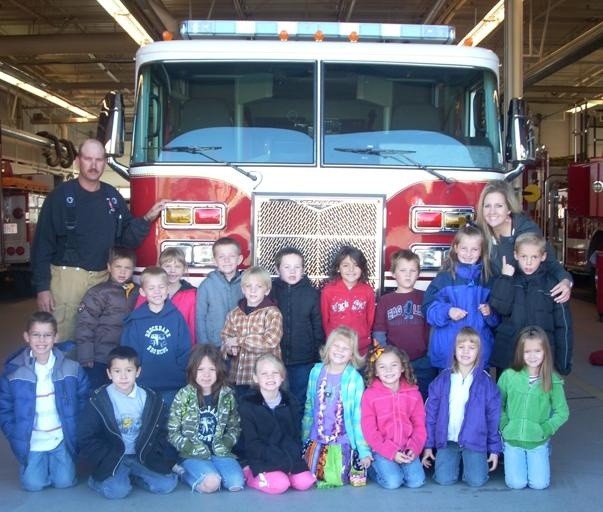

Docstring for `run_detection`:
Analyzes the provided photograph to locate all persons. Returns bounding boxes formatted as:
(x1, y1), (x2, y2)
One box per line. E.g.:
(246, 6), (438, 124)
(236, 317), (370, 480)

(30, 139), (172, 346)
(0, 312), (93, 492)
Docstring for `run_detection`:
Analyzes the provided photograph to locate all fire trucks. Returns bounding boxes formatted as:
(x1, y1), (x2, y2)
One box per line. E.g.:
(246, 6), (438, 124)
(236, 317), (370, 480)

(535, 158), (603, 304)
(0, 160), (65, 283)
(93, 18), (532, 296)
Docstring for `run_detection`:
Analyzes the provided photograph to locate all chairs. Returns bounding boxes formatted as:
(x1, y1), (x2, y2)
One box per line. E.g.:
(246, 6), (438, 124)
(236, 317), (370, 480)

(390, 103), (443, 135)
(169, 99), (228, 143)
